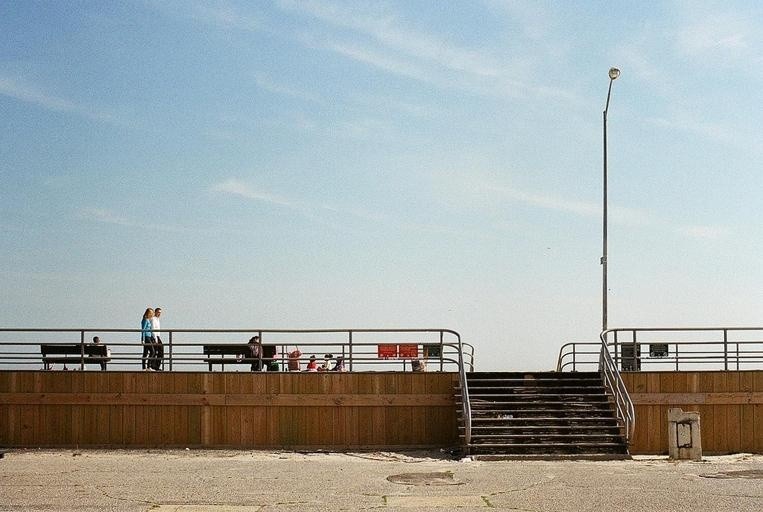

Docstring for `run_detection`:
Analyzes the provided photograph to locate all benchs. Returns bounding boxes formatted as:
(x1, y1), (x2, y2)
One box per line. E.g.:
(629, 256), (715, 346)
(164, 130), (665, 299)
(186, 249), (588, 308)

(202, 343), (276, 372)
(39, 342), (111, 371)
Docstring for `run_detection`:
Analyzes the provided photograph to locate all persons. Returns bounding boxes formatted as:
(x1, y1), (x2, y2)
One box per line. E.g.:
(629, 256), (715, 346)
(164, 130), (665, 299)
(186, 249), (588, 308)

(140, 308), (156, 371)
(317, 354), (331, 372)
(149, 307), (164, 371)
(330, 356), (343, 370)
(87, 336), (111, 371)
(244, 337), (255, 358)
(305, 354), (316, 372)
(247, 336), (264, 372)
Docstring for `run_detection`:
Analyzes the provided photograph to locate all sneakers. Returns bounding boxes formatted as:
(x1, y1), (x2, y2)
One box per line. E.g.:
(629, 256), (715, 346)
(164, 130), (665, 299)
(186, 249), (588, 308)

(142, 367), (161, 372)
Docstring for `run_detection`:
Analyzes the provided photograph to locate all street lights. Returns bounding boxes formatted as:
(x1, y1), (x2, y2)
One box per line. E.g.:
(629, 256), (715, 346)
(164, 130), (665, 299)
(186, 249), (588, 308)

(593, 62), (624, 374)
